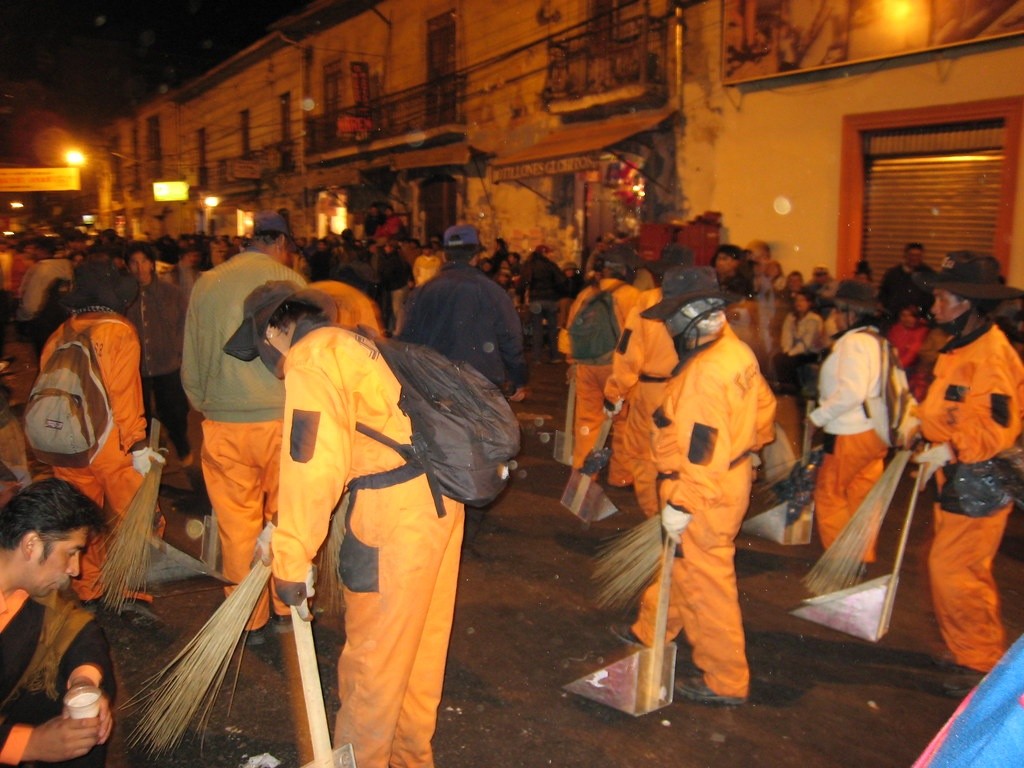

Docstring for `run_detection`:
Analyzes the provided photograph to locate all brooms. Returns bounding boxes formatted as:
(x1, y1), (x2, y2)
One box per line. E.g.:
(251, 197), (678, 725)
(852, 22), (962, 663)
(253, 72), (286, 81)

(319, 492), (351, 599)
(761, 421), (796, 484)
(799, 450), (911, 596)
(91, 417), (165, 615)
(590, 513), (663, 609)
(116, 556), (272, 762)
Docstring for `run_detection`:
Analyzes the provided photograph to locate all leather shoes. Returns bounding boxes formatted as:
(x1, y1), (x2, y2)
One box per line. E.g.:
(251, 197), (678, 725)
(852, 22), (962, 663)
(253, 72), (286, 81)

(676, 675), (747, 706)
(610, 624), (644, 647)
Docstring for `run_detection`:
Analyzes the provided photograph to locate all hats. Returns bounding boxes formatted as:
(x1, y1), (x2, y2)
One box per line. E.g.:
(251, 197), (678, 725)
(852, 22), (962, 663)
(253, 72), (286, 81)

(641, 264), (736, 319)
(245, 211), (295, 244)
(644, 243), (695, 278)
(443, 224), (488, 253)
(818, 280), (882, 317)
(226, 279), (326, 360)
(921, 250), (1024, 302)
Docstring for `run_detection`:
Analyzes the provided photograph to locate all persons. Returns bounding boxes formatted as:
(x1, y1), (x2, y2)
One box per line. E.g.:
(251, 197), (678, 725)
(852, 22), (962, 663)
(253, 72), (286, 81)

(800, 281), (909, 576)
(0, 478), (117, 768)
(0, 223), (309, 346)
(122, 243), (191, 470)
(888, 304), (927, 375)
(706, 240), (882, 406)
(476, 230), (694, 518)
(877, 242), (938, 320)
(614, 264), (777, 704)
(42, 253), (164, 608)
(911, 252), (1024, 695)
(0, 395), (31, 492)
(391, 225), (527, 403)
(220, 282), (465, 767)
(179, 216), (309, 645)
(297, 201), (447, 334)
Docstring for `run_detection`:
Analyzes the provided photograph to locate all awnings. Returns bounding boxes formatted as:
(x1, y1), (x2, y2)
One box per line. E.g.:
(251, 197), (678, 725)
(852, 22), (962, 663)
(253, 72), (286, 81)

(306, 144), (494, 218)
(493, 105), (675, 207)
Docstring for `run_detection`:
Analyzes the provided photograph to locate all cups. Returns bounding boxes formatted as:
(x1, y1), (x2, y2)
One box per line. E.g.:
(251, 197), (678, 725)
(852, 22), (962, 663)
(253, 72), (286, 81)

(62, 684), (103, 719)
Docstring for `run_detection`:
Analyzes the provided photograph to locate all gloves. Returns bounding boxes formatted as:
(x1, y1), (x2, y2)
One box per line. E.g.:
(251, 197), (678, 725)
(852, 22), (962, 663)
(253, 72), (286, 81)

(602, 398), (624, 420)
(910, 445), (953, 492)
(660, 506), (692, 542)
(293, 569), (315, 621)
(251, 521), (277, 569)
(130, 446), (167, 477)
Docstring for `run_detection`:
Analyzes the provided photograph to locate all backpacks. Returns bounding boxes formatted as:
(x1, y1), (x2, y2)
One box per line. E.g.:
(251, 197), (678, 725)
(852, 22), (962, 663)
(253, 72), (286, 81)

(570, 280), (629, 359)
(24, 315), (113, 466)
(297, 324), (522, 508)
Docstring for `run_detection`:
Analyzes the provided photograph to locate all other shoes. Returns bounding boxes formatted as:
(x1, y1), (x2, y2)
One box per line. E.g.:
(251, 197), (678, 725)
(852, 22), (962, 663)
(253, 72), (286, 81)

(943, 666), (986, 689)
(99, 595), (165, 633)
(605, 482), (635, 496)
(179, 451), (194, 468)
